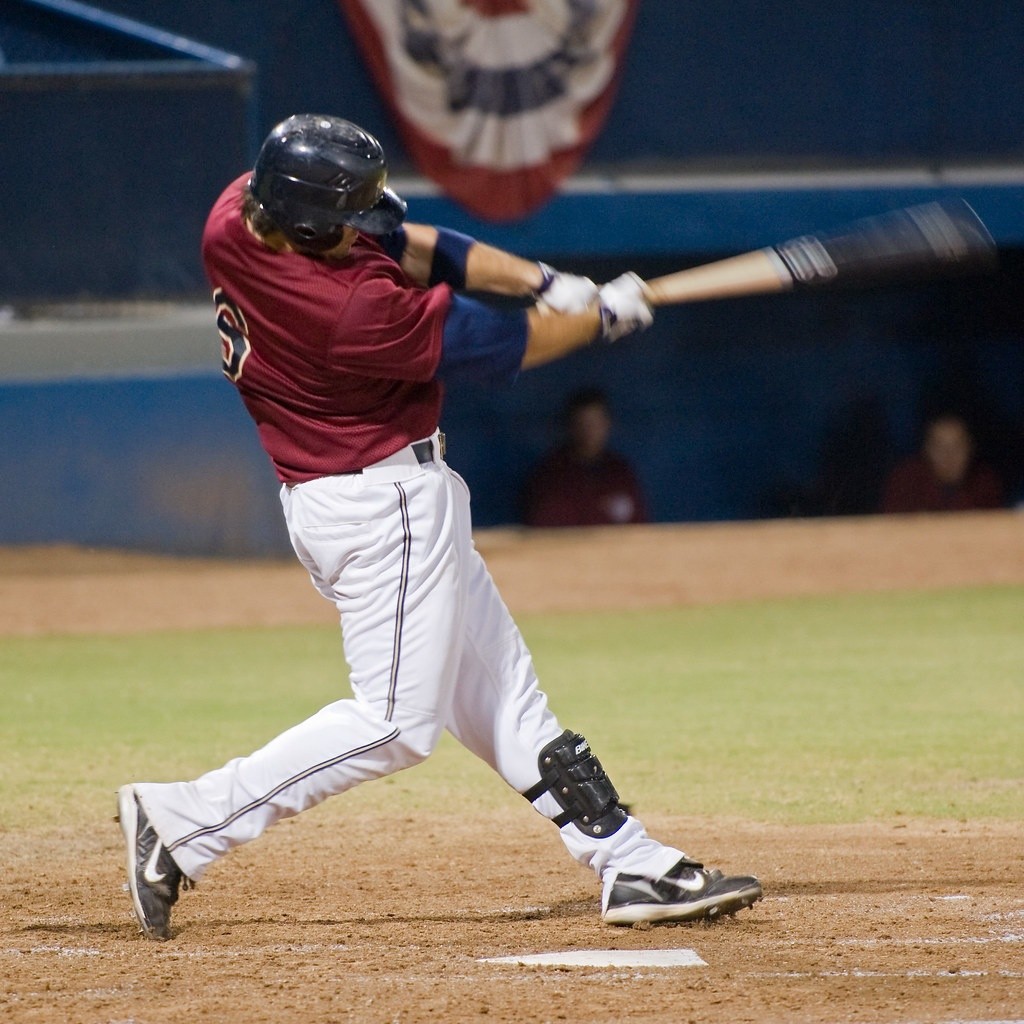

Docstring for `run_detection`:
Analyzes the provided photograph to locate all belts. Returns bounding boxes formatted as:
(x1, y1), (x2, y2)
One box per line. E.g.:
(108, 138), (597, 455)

(341, 432), (449, 475)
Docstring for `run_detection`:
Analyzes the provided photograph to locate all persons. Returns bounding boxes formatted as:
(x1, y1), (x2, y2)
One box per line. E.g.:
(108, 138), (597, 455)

(522, 387), (649, 526)
(877, 403), (1003, 513)
(117, 114), (763, 941)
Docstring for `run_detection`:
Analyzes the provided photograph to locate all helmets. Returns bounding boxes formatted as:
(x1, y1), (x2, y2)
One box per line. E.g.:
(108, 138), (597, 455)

(248, 112), (409, 251)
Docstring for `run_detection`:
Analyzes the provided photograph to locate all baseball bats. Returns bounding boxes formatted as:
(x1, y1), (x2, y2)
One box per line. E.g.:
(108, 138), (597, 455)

(646, 183), (1024, 305)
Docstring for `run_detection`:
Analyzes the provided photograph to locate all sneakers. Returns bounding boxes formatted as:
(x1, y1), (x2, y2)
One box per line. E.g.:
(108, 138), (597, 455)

(117, 784), (188, 941)
(598, 855), (762, 924)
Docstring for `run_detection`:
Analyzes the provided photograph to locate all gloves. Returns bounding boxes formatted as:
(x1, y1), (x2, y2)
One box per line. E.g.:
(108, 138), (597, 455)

(597, 269), (656, 343)
(533, 258), (598, 317)
